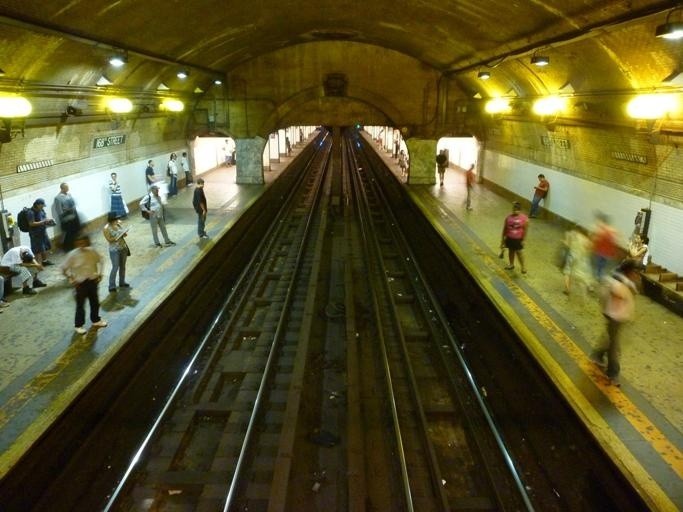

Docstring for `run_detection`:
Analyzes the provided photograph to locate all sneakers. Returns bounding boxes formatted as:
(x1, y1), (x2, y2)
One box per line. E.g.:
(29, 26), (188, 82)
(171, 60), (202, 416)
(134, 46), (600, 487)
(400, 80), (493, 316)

(34, 280), (46, 287)
(23, 286), (36, 294)
(42, 259), (54, 265)
(75, 327), (86, 334)
(92, 321), (107, 327)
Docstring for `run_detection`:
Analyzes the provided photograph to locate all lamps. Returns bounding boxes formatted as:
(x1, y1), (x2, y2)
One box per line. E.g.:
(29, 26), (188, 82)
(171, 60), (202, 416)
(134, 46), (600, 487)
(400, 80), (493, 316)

(529, 44), (549, 67)
(654, 7), (682, 40)
(109, 42), (128, 65)
(477, 61), (490, 79)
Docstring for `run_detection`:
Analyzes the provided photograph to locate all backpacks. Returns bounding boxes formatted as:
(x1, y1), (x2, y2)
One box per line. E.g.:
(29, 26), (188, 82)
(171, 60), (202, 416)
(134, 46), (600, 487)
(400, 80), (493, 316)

(18, 207), (29, 232)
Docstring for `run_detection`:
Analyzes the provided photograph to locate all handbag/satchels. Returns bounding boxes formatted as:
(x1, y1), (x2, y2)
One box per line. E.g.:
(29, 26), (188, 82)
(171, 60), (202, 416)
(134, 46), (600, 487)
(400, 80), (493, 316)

(142, 205), (149, 219)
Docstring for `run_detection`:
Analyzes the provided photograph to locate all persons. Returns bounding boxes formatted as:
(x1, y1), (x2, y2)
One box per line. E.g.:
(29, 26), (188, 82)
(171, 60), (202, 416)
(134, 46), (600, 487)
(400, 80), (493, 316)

(320, 125), (324, 141)
(395, 141), (400, 158)
(222, 139), (232, 167)
(501, 202), (528, 274)
(464, 164), (476, 210)
(559, 216), (649, 387)
(286, 137), (290, 154)
(398, 150), (407, 175)
(436, 149), (447, 185)
(0, 153), (208, 335)
(529, 174), (550, 218)
(378, 130), (384, 148)
(300, 129), (303, 142)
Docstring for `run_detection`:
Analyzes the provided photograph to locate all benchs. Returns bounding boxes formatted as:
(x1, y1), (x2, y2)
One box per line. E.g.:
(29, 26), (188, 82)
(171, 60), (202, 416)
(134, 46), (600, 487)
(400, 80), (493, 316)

(0, 265), (19, 297)
(638, 263), (682, 317)
